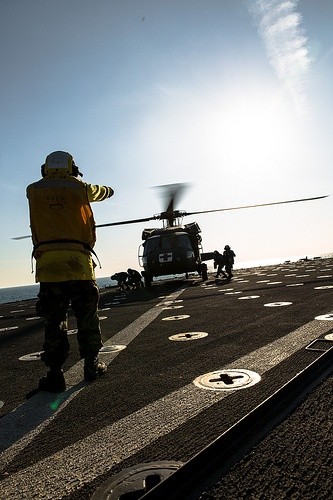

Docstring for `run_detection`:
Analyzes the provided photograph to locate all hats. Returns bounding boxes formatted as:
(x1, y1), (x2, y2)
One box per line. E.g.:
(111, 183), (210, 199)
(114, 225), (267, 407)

(43, 151), (75, 176)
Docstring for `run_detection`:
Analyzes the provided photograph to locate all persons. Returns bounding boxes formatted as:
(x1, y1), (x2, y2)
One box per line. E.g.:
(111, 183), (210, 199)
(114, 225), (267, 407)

(213, 250), (228, 278)
(25, 151), (114, 393)
(110, 272), (130, 291)
(127, 268), (143, 290)
(222, 245), (236, 278)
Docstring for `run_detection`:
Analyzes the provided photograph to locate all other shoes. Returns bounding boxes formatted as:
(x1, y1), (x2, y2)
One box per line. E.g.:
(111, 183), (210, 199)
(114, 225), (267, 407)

(37, 367), (66, 394)
(82, 358), (108, 383)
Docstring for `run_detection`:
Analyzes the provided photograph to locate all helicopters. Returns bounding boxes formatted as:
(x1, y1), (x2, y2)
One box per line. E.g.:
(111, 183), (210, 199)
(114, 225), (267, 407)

(12, 182), (329, 289)
